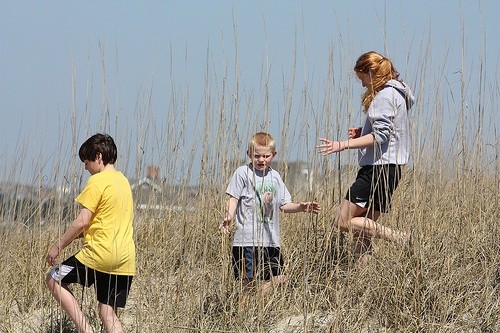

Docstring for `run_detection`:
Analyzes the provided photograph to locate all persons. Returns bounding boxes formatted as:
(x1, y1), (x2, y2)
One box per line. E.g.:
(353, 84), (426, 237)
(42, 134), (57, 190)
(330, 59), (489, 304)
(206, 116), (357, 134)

(46, 134), (136, 333)
(315, 52), (414, 268)
(219, 133), (320, 294)
